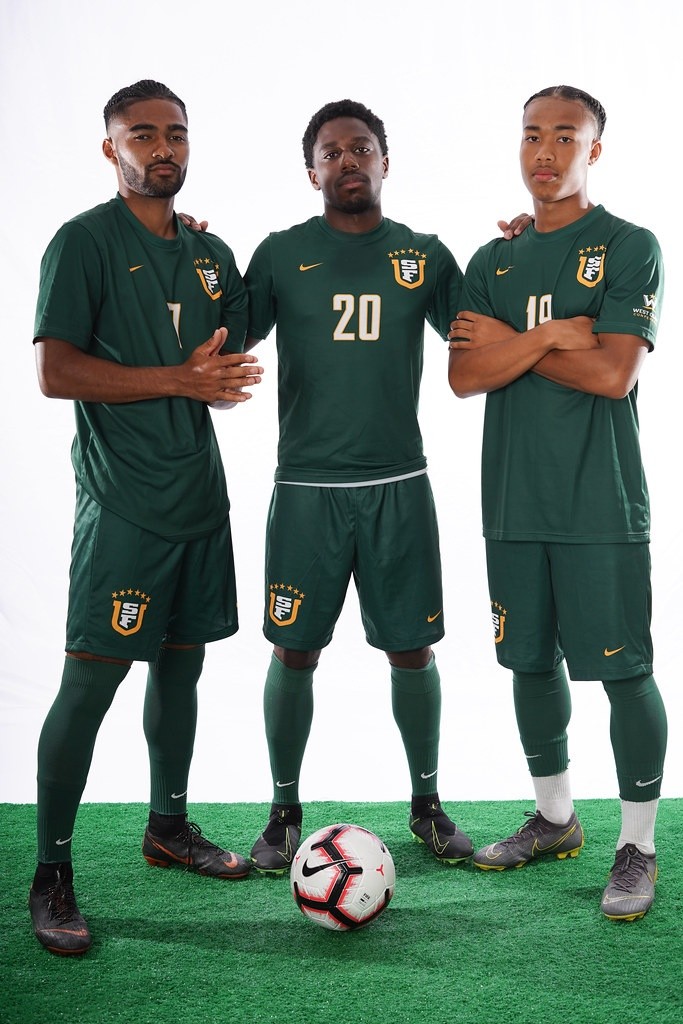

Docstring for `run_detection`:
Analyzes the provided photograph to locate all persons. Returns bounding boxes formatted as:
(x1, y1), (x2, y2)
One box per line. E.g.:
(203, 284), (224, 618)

(173, 97), (532, 876)
(27, 79), (254, 952)
(445, 83), (666, 923)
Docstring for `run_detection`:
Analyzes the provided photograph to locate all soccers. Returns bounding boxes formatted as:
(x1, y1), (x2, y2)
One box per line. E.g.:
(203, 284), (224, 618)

(291, 823), (399, 931)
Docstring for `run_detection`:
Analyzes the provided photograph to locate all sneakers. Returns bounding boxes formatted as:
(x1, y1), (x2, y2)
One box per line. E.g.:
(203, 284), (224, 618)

(599, 843), (657, 921)
(141, 821), (250, 878)
(472, 809), (583, 871)
(408, 793), (474, 865)
(250, 803), (302, 875)
(28, 864), (91, 956)
